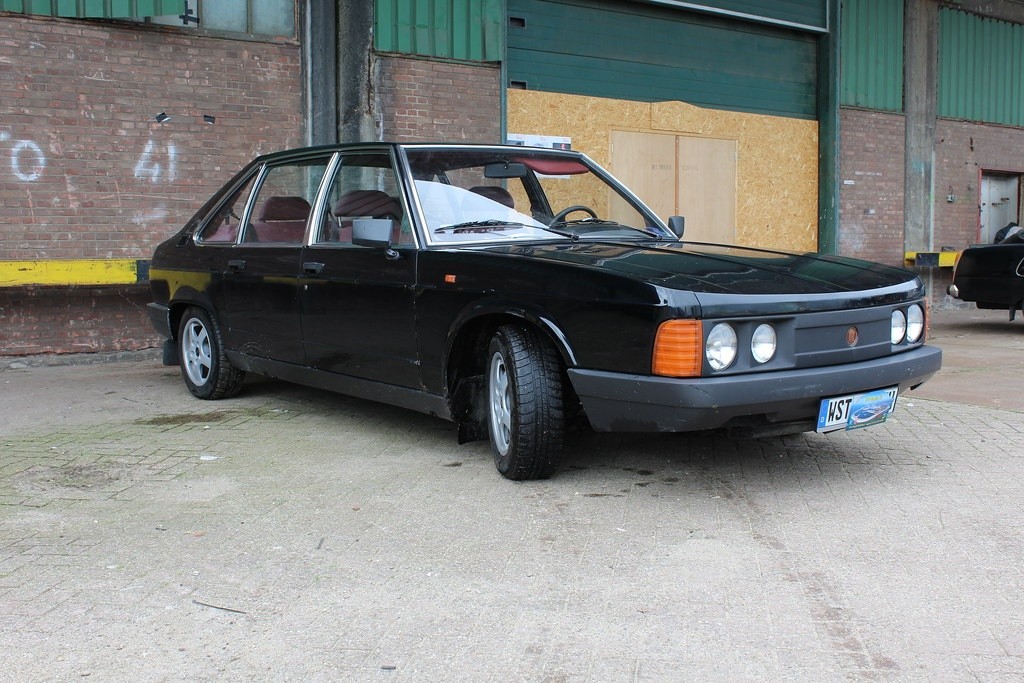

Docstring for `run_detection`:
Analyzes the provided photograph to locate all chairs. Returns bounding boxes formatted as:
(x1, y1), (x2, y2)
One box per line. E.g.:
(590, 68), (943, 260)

(452, 186), (524, 243)
(205, 191), (405, 246)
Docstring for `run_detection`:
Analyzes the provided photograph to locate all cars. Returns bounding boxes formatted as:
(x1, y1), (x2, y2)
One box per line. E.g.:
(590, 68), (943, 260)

(946, 243), (1024, 323)
(147, 140), (944, 482)
